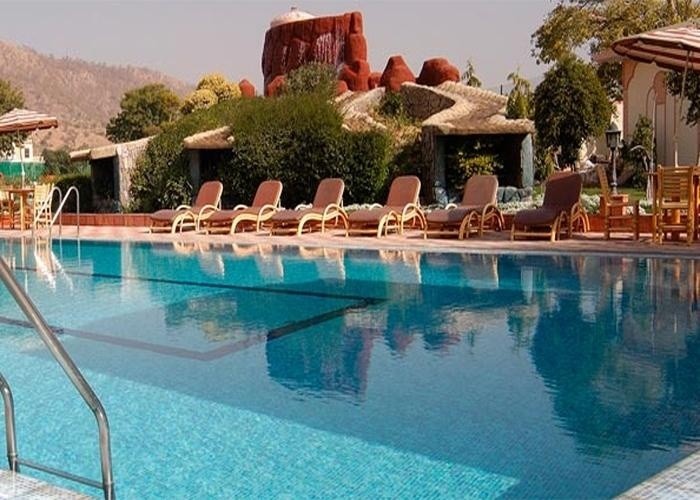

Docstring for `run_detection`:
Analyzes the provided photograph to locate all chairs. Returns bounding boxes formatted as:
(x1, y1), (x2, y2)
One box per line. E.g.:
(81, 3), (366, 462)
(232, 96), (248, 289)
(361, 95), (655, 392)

(462, 251), (499, 291)
(298, 245), (347, 289)
(150, 180), (224, 234)
(379, 249), (421, 300)
(174, 241), (225, 280)
(346, 175), (428, 240)
(596, 164), (700, 247)
(515, 254), (699, 325)
(232, 242), (285, 284)
(509, 172), (590, 242)
(269, 178), (350, 237)
(0, 237), (74, 290)
(206, 180), (284, 234)
(0, 182), (55, 230)
(424, 175), (506, 240)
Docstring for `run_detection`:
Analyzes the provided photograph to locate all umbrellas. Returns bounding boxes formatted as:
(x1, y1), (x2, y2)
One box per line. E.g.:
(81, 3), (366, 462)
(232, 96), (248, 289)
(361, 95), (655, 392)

(0, 107), (54, 171)
(610, 22), (699, 168)
